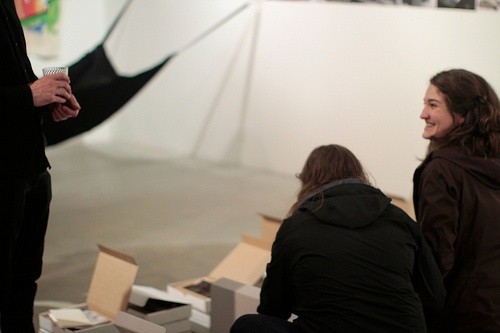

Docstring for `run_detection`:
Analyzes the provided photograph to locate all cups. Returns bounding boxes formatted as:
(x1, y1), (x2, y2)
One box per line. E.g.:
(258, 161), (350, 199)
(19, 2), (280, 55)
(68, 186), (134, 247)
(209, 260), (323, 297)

(42, 65), (72, 112)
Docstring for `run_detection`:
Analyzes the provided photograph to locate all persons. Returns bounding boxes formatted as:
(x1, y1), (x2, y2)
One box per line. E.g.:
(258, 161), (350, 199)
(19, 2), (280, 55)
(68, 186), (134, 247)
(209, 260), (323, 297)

(0, 0), (81, 333)
(413, 69), (500, 333)
(228, 145), (427, 333)
